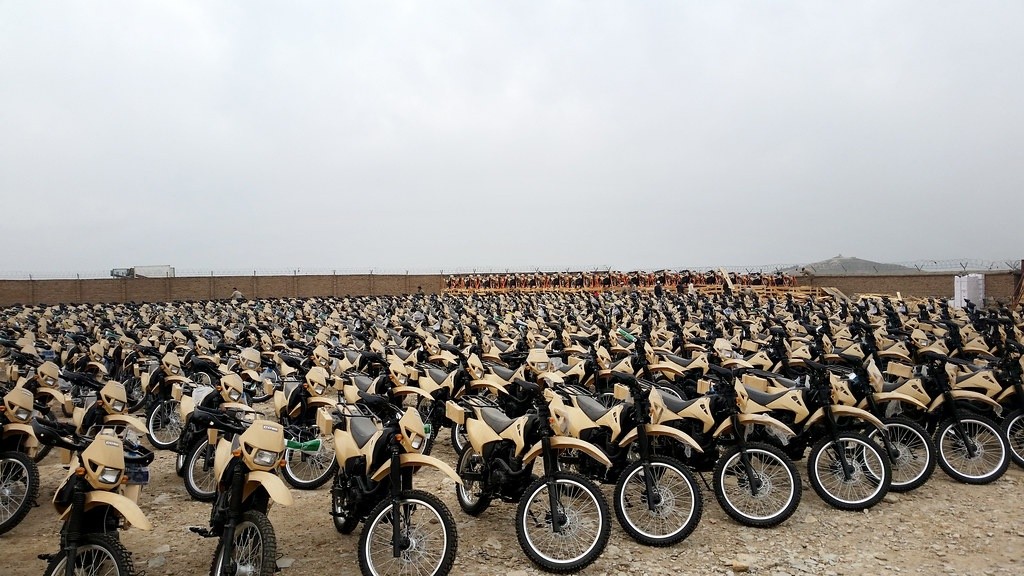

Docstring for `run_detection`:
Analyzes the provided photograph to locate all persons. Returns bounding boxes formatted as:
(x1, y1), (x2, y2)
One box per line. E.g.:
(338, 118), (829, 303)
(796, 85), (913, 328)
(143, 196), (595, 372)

(800, 268), (814, 286)
(745, 286), (754, 295)
(415, 286), (425, 297)
(723, 278), (728, 292)
(676, 282), (684, 293)
(228, 287), (244, 298)
(628, 282), (636, 293)
(620, 282), (627, 295)
(689, 280), (693, 292)
(654, 281), (663, 296)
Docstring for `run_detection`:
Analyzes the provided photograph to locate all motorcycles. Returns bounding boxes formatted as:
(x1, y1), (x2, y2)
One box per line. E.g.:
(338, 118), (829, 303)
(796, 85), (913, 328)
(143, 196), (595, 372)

(1, 264), (1024, 576)
